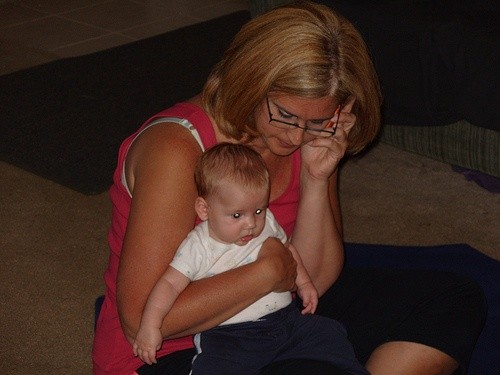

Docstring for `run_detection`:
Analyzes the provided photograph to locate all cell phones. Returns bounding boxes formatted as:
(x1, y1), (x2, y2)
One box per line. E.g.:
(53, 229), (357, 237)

(325, 105), (341, 129)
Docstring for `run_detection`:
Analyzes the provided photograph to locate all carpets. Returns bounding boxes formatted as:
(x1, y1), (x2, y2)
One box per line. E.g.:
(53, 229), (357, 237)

(0, 11), (252, 197)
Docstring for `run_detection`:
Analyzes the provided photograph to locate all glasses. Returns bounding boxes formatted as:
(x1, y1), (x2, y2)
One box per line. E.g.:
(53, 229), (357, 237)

(266, 94), (341, 138)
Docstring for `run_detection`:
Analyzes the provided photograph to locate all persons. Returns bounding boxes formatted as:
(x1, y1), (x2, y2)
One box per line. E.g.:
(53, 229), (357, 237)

(91, 5), (487, 375)
(132, 142), (370, 375)
